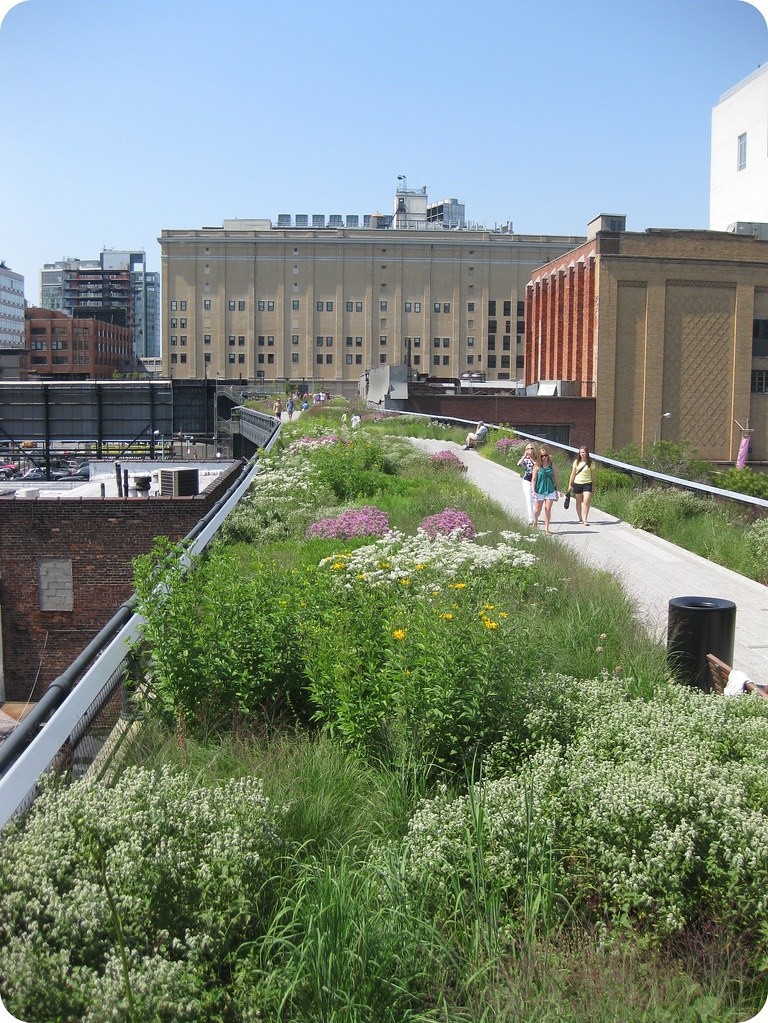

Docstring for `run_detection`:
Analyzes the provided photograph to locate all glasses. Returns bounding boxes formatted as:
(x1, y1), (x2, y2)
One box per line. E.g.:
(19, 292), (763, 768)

(541, 454), (549, 457)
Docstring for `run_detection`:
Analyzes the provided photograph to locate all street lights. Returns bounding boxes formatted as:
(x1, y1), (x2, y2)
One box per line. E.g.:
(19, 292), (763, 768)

(515, 381), (522, 395)
(153, 429), (164, 460)
(652, 412), (671, 470)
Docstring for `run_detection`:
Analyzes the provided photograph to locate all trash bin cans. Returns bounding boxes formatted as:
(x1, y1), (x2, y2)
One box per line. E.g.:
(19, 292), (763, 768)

(257, 370), (264, 377)
(666, 596), (737, 693)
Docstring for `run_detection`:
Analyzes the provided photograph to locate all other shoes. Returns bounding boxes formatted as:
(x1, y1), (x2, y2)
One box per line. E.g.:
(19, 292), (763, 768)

(463, 447), (469, 450)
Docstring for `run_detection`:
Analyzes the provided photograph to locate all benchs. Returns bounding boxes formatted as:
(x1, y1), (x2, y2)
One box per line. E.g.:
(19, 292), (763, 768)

(470, 432), (487, 449)
(705, 653), (768, 699)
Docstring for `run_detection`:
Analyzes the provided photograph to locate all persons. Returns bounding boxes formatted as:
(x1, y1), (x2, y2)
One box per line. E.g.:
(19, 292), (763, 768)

(293, 390), (330, 405)
(286, 398), (293, 421)
(516, 445), (538, 527)
(303, 401), (308, 410)
(273, 399), (283, 420)
(341, 412), (361, 428)
(216, 452), (221, 459)
(569, 446), (595, 526)
(532, 449), (563, 533)
(462, 421), (488, 450)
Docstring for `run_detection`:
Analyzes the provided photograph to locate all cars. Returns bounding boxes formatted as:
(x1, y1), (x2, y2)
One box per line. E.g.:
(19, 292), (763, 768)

(0, 451), (89, 482)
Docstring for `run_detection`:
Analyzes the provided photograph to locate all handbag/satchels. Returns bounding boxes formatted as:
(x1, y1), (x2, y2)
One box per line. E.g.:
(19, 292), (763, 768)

(564, 492), (570, 509)
